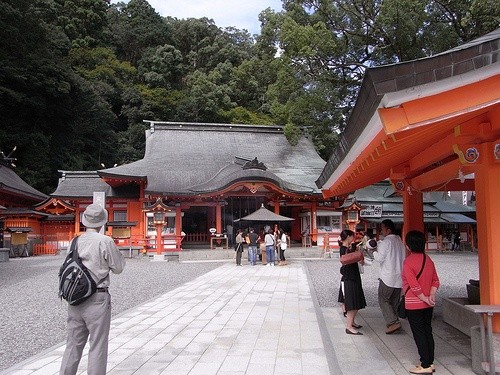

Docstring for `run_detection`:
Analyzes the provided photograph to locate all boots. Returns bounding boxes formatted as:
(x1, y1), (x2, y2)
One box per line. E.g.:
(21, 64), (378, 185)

(278, 261), (283, 265)
(283, 260), (287, 265)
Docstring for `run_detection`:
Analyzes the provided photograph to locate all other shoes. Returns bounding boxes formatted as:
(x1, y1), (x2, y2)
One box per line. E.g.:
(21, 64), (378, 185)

(352, 324), (363, 329)
(346, 328), (363, 335)
(266, 263), (270, 266)
(271, 263), (274, 266)
(344, 312), (347, 317)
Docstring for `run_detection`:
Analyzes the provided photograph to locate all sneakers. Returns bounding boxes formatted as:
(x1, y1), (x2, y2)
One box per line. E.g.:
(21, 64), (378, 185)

(409, 365), (432, 375)
(416, 363), (435, 372)
(385, 322), (400, 333)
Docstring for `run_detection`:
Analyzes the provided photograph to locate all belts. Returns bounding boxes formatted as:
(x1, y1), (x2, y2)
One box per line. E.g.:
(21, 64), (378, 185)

(97, 288), (105, 292)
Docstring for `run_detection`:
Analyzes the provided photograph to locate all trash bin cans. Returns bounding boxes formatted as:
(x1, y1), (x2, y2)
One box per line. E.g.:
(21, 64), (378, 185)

(465, 304), (500, 375)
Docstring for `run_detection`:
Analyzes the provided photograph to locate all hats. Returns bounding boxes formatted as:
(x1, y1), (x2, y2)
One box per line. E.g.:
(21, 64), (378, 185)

(82, 204), (108, 227)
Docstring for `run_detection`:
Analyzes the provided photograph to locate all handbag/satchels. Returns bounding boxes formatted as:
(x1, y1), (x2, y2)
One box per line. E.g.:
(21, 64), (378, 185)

(340, 246), (363, 265)
(397, 295), (407, 319)
(246, 233), (250, 243)
(58, 234), (97, 306)
(274, 242), (276, 250)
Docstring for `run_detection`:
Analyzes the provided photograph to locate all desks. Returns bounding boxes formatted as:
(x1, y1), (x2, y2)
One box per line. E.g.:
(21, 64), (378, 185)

(210, 236), (229, 252)
(463, 304), (500, 375)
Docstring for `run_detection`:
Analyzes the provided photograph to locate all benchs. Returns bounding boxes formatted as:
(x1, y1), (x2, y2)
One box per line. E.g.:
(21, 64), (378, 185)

(118, 245), (144, 259)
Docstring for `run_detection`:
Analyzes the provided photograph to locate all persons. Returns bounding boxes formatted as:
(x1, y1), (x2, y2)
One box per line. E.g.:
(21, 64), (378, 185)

(448, 228), (461, 251)
(361, 231), (374, 261)
(401, 230), (440, 375)
(320, 233), (331, 258)
(234, 228), (287, 266)
(59, 204), (125, 375)
(226, 224), (233, 247)
(366, 219), (406, 335)
(264, 225), (270, 238)
(338, 230), (364, 335)
